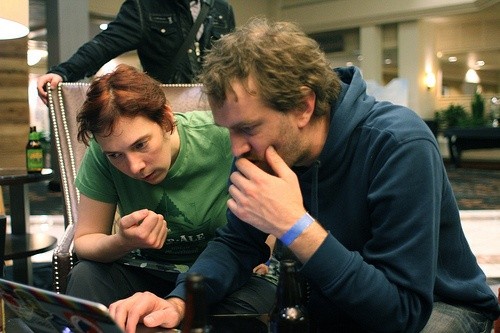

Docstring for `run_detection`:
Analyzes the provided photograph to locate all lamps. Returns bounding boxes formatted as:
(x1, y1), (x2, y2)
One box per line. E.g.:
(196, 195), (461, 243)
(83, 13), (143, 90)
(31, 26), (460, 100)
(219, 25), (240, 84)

(426, 72), (439, 92)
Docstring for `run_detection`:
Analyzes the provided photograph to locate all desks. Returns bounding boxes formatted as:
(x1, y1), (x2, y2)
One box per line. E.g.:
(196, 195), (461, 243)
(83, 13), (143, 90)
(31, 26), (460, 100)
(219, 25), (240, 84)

(0, 233), (56, 294)
(0, 166), (59, 287)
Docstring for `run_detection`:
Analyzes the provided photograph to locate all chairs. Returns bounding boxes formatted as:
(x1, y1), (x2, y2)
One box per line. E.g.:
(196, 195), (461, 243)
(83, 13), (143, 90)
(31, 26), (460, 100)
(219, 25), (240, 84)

(45, 78), (220, 297)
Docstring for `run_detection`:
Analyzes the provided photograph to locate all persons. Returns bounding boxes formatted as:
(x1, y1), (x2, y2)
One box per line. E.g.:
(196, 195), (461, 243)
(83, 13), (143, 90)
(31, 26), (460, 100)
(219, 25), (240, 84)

(58, 65), (281, 333)
(37, 0), (239, 107)
(104, 18), (500, 332)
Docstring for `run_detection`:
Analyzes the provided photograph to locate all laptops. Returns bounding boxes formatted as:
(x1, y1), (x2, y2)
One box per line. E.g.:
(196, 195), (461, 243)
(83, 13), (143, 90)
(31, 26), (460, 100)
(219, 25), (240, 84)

(0, 278), (155, 333)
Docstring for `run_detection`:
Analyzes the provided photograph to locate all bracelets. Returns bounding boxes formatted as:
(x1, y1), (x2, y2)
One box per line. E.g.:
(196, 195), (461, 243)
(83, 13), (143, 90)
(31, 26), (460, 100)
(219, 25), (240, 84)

(280, 212), (314, 248)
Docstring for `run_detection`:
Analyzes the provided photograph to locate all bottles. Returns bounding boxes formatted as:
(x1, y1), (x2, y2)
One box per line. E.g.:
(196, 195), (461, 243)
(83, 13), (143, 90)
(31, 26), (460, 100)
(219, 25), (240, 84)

(266, 259), (311, 332)
(25, 125), (45, 174)
(178, 274), (212, 332)
